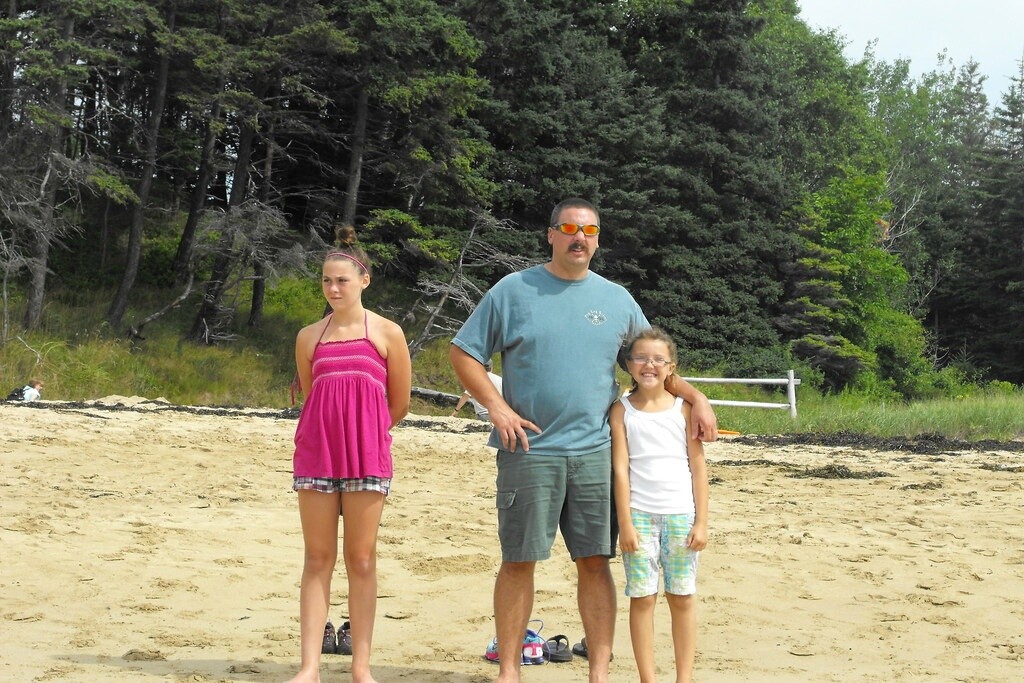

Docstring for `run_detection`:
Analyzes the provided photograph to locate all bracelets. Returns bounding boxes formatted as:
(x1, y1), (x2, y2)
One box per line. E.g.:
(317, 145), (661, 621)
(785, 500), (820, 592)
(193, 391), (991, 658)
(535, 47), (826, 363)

(455, 409), (459, 412)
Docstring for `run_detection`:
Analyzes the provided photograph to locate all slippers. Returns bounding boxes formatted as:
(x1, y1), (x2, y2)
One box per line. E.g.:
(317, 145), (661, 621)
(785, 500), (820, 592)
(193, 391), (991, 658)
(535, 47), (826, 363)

(573, 637), (613, 662)
(543, 635), (571, 661)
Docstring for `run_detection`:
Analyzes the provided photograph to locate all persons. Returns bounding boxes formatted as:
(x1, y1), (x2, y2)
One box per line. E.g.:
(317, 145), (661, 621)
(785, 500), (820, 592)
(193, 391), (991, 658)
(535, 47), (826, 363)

(608, 331), (710, 683)
(449, 197), (717, 683)
(286, 227), (412, 683)
(451, 357), (503, 423)
(21, 380), (43, 402)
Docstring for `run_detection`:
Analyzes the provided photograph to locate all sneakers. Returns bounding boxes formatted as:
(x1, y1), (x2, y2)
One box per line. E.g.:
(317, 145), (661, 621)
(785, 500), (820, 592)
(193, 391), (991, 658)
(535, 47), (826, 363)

(322, 621), (337, 653)
(337, 622), (352, 655)
(521, 628), (544, 664)
(485, 635), (524, 665)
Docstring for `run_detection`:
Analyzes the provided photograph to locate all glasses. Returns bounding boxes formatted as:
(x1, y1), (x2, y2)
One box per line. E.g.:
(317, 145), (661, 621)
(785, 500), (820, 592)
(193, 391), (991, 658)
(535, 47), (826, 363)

(551, 223), (600, 236)
(627, 356), (671, 367)
